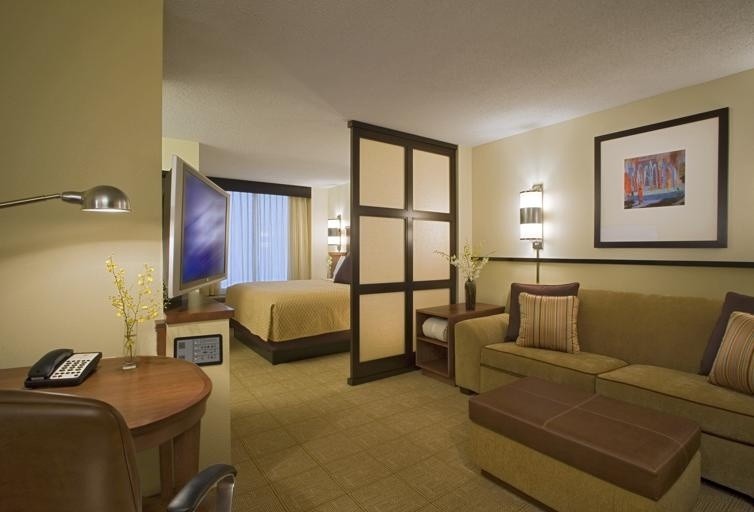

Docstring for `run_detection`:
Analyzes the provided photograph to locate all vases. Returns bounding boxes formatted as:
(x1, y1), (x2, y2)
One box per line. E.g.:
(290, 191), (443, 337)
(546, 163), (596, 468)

(465, 281), (477, 309)
(121, 321), (136, 367)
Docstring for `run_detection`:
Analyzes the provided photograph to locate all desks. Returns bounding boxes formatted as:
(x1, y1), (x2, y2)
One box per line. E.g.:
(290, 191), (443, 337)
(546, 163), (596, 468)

(0, 355), (211, 495)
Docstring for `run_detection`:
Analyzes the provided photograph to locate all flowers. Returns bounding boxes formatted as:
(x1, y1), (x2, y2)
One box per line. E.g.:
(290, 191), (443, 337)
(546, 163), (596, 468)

(105, 255), (164, 321)
(432, 243), (499, 279)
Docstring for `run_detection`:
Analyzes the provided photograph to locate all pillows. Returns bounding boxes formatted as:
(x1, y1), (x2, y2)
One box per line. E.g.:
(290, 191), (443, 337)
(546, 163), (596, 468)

(709, 311), (754, 389)
(516, 295), (581, 352)
(333, 251), (353, 283)
(506, 283), (580, 342)
(698, 291), (754, 374)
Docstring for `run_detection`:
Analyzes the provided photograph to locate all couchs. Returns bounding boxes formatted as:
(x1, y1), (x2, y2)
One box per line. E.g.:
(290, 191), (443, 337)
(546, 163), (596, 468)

(454, 288), (754, 499)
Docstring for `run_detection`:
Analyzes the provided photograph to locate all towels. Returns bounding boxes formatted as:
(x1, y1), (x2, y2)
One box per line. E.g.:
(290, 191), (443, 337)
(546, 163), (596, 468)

(421, 318), (448, 341)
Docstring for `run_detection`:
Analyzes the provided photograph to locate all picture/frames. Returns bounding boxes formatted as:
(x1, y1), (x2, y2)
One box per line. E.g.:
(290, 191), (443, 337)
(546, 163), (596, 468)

(594, 110), (729, 248)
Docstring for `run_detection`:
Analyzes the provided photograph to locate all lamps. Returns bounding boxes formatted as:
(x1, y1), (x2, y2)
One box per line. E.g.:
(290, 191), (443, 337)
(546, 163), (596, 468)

(1, 186), (131, 215)
(327, 219), (341, 252)
(519, 189), (542, 240)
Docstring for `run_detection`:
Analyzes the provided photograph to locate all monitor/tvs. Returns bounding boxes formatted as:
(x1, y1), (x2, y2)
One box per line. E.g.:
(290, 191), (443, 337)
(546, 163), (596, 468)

(161, 154), (231, 299)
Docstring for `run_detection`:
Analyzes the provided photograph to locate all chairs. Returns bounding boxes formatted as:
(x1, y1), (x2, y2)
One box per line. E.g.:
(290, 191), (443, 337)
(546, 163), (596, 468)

(0, 389), (236, 511)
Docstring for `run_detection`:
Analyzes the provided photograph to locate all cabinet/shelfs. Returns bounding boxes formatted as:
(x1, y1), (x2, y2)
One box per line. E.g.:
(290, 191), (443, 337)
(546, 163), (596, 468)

(416, 301), (504, 384)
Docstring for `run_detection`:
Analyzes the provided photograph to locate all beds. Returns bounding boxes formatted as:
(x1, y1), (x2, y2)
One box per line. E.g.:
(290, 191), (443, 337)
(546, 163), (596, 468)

(227, 279), (351, 365)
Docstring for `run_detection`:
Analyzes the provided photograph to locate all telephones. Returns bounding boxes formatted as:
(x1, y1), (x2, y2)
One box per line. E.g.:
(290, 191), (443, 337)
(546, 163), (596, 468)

(24, 349), (102, 388)
